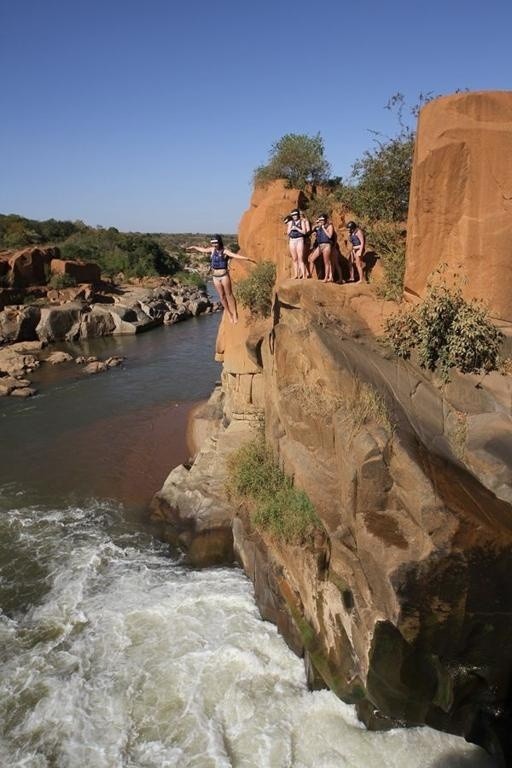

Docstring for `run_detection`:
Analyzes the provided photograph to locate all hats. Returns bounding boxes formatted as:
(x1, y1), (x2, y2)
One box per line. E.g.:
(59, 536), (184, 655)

(211, 234), (222, 243)
(284, 209), (300, 224)
(318, 215), (327, 220)
(347, 221), (356, 229)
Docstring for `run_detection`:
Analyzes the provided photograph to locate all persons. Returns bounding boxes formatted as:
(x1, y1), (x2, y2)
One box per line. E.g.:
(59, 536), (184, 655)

(284, 209), (365, 284)
(186, 234), (257, 325)
